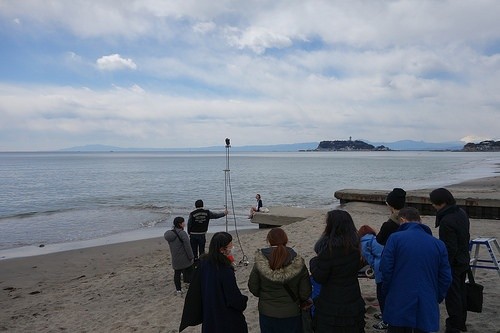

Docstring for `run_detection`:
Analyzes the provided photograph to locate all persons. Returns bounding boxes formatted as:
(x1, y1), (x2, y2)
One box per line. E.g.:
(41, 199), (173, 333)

(165, 216), (195, 295)
(375, 187), (406, 245)
(378, 207), (452, 333)
(248, 228), (312, 333)
(358, 225), (391, 330)
(429, 188), (470, 333)
(186, 199), (228, 258)
(305, 256), (321, 317)
(248, 194), (262, 219)
(308, 209), (367, 333)
(179, 232), (249, 332)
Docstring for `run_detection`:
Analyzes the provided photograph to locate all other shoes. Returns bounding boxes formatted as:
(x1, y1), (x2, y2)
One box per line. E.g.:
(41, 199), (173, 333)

(372, 313), (388, 329)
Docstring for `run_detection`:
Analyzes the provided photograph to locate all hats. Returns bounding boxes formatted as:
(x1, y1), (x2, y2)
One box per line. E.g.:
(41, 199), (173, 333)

(386, 188), (406, 209)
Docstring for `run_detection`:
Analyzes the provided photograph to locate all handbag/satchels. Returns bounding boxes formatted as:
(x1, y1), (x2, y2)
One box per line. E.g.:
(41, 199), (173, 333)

(466, 265), (485, 313)
(302, 308), (314, 333)
